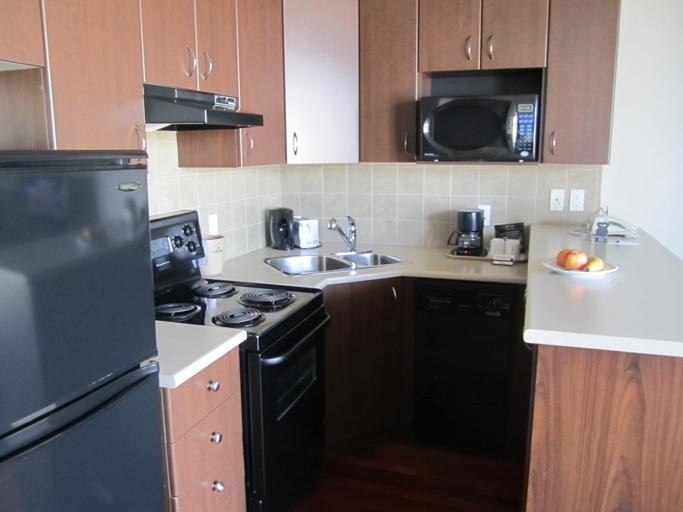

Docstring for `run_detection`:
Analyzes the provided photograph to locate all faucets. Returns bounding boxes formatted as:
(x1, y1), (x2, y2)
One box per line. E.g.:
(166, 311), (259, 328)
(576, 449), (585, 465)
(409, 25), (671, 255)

(327, 215), (358, 252)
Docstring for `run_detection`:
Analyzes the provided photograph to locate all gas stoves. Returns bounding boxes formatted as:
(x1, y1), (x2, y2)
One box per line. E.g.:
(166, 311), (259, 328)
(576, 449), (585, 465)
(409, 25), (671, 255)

(154, 278), (323, 353)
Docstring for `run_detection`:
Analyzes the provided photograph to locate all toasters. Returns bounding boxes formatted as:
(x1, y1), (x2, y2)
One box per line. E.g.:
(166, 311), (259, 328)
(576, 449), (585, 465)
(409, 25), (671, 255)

(294, 216), (320, 249)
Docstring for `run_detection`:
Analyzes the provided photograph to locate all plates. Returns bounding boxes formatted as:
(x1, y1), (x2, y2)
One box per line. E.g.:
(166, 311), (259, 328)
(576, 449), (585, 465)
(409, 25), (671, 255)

(542, 257), (618, 279)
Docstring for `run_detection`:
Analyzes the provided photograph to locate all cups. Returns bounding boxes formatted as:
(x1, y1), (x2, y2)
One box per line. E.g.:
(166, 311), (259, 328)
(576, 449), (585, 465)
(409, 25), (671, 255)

(507, 239), (521, 260)
(489, 239), (505, 257)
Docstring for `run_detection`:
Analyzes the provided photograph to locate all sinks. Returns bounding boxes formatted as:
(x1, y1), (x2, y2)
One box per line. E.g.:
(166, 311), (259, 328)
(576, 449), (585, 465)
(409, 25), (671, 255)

(329, 248), (402, 269)
(262, 254), (359, 278)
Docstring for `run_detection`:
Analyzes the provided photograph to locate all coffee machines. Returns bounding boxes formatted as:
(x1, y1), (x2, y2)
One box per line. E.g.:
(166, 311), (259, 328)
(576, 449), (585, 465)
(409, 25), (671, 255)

(446, 209), (484, 256)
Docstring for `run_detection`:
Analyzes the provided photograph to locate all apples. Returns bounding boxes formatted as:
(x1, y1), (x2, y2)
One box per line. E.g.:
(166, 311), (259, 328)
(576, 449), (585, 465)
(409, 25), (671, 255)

(566, 251), (588, 270)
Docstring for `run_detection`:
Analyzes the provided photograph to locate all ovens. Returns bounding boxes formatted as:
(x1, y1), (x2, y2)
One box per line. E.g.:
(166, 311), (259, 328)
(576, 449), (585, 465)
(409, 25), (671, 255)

(239, 303), (331, 512)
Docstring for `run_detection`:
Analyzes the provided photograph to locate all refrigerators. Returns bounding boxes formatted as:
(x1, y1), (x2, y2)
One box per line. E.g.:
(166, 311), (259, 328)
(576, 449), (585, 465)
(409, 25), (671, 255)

(0, 150), (166, 512)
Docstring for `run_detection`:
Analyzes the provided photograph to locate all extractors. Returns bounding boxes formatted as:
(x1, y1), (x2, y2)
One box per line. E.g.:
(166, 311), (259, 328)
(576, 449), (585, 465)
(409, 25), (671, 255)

(143, 83), (263, 133)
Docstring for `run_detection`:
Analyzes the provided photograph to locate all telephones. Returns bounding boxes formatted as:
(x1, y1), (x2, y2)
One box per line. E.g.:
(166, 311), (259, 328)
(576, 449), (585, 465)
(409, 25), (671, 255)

(589, 205), (636, 237)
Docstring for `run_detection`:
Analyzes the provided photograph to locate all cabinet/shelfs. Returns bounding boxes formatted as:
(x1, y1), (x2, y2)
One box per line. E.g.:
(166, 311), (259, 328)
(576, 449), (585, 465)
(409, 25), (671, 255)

(540, 0), (620, 165)
(358, 0), (418, 162)
(324, 276), (411, 444)
(177, 0), (286, 167)
(412, 274), (529, 455)
(281, 0), (359, 164)
(159, 346), (247, 512)
(139, 0), (240, 97)
(520, 346), (682, 512)
(417, 0), (549, 72)
(0, 0), (147, 151)
(0, 0), (45, 70)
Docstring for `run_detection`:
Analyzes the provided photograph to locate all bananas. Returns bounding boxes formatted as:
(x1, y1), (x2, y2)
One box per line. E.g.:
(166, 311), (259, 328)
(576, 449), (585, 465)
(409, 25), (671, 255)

(584, 257), (603, 272)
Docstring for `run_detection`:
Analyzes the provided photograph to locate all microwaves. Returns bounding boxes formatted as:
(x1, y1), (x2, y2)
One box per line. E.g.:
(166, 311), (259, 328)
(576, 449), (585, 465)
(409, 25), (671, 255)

(415, 93), (539, 164)
